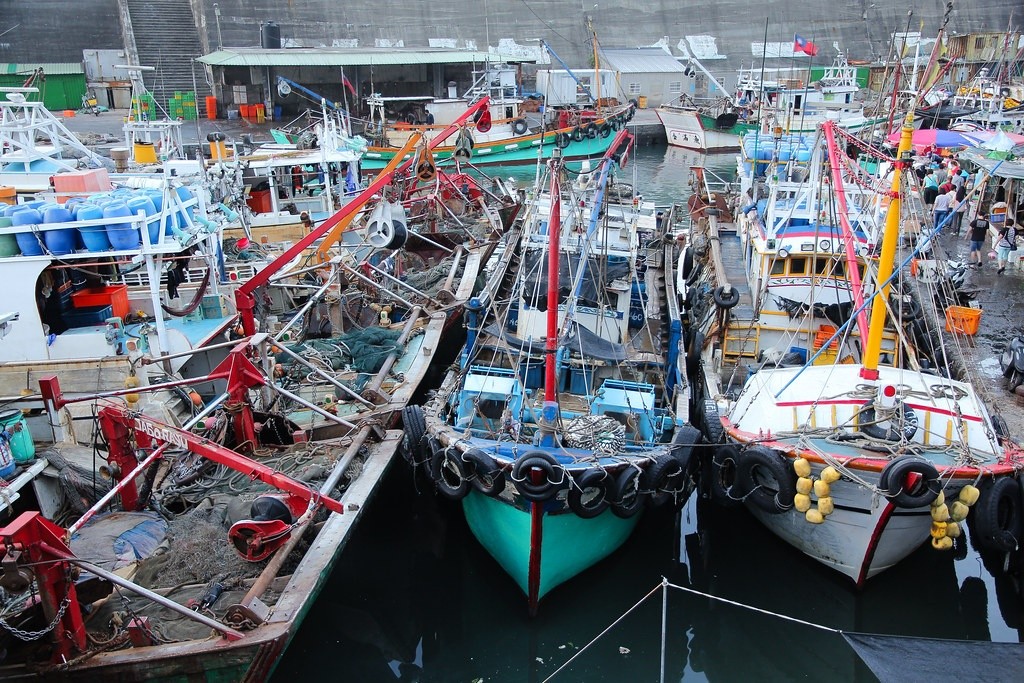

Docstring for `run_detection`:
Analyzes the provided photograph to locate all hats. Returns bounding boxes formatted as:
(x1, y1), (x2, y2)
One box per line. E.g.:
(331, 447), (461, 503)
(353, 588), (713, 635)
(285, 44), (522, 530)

(926, 152), (931, 158)
(988, 251), (996, 260)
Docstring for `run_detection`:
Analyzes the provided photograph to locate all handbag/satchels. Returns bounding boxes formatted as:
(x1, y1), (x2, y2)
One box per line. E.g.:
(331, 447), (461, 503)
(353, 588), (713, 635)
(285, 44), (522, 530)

(1010, 244), (1017, 250)
(930, 181), (938, 191)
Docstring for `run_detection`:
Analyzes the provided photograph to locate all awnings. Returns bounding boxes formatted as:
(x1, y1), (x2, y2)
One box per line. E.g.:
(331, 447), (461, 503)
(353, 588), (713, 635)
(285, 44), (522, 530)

(194, 49), (536, 66)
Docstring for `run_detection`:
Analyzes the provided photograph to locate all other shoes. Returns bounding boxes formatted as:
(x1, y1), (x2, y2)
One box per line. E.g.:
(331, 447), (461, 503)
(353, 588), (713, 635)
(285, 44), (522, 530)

(968, 262), (975, 265)
(977, 262), (982, 267)
(997, 267), (1005, 276)
(951, 232), (958, 235)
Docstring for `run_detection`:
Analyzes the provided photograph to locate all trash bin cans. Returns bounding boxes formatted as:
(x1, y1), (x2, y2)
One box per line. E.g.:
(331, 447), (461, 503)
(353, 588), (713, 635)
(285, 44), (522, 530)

(274, 104), (282, 122)
(639, 96), (648, 108)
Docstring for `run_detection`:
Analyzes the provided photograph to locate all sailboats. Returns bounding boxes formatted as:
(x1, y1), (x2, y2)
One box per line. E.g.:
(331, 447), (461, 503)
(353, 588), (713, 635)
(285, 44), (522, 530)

(0, 2), (1024, 683)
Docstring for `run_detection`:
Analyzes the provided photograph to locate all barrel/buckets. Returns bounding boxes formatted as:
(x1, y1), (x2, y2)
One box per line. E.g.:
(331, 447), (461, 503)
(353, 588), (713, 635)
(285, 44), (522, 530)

(0, 433), (16, 477)
(0, 185), (194, 258)
(261, 20), (281, 49)
(538, 105), (545, 113)
(0, 408), (36, 462)
(639, 95), (648, 110)
(740, 133), (828, 182)
(236, 237), (250, 253)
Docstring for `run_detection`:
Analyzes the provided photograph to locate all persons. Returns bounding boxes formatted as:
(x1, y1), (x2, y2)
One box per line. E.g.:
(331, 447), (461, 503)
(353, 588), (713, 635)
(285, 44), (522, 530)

(394, 108), (434, 131)
(965, 210), (990, 267)
(910, 150), (969, 236)
(992, 219), (1019, 274)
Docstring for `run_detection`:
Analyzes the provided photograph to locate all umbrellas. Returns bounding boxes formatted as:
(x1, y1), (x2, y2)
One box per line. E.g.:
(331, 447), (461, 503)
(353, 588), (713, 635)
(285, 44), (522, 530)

(883, 127), (1024, 164)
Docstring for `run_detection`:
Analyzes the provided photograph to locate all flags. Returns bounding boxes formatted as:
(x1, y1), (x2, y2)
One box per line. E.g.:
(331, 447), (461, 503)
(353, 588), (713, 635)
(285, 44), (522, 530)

(343, 75), (355, 94)
(792, 33), (819, 56)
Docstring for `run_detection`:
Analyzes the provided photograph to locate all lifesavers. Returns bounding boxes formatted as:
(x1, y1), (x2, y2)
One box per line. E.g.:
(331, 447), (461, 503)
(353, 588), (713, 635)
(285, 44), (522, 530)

(855, 396), (920, 443)
(511, 117), (529, 134)
(972, 473), (1024, 557)
(397, 395), (799, 520)
(876, 452), (945, 513)
(680, 246), (706, 369)
(713, 283), (741, 310)
(554, 97), (638, 150)
(892, 295), (920, 321)
